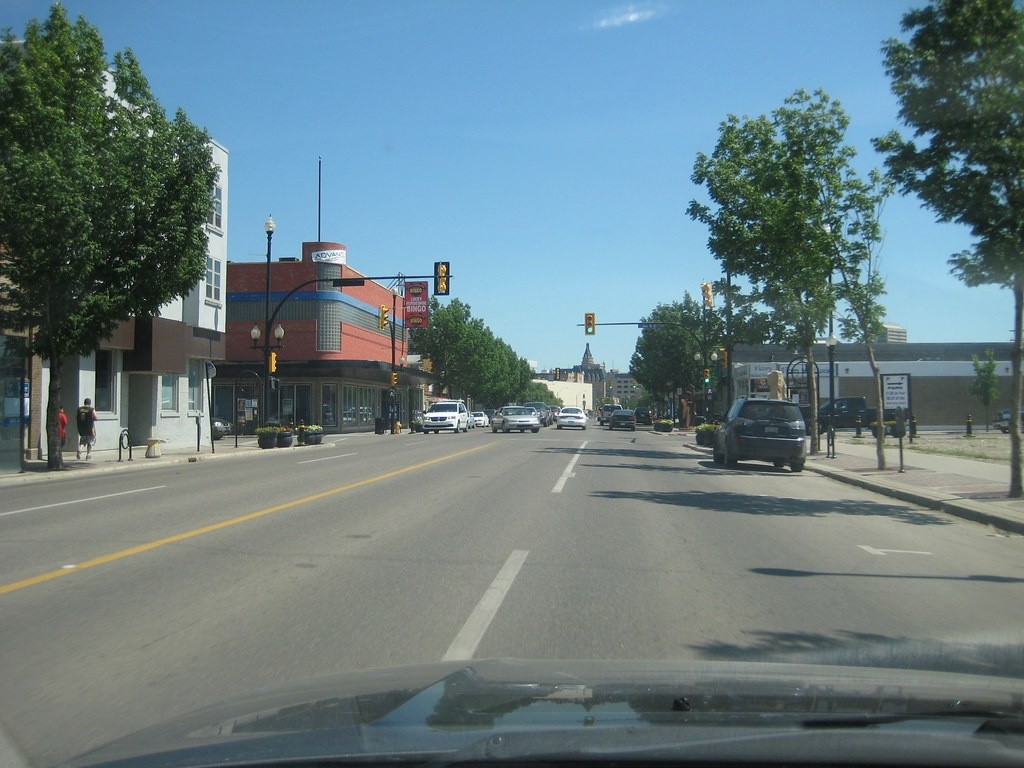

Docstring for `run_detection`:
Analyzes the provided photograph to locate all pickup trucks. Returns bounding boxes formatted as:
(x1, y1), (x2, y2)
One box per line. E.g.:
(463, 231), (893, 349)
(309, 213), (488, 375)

(819, 396), (896, 432)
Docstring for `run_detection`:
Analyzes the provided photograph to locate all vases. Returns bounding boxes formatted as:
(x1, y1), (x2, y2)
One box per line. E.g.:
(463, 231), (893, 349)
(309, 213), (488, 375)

(654, 422), (672, 432)
(704, 431), (714, 446)
(890, 425), (906, 438)
(277, 432), (292, 446)
(257, 432), (277, 448)
(312, 431), (324, 443)
(696, 432), (705, 445)
(873, 425), (887, 438)
(304, 431), (316, 445)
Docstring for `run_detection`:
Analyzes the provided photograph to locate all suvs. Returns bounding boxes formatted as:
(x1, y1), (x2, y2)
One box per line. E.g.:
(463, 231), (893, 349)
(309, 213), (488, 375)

(598, 404), (623, 426)
(713, 398), (823, 472)
(422, 398), (470, 434)
(524, 402), (550, 427)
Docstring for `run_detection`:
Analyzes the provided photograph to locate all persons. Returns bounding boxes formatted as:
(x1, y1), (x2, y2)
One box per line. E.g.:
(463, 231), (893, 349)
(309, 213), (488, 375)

(77, 398), (97, 460)
(58, 405), (68, 447)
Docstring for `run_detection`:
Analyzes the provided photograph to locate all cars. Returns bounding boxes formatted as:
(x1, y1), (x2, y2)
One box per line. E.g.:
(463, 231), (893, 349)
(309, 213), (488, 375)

(555, 406), (589, 430)
(211, 417), (231, 440)
(992, 407), (1024, 434)
(634, 407), (653, 424)
(549, 404), (561, 422)
(609, 409), (636, 431)
(490, 406), (541, 433)
(467, 409), (496, 429)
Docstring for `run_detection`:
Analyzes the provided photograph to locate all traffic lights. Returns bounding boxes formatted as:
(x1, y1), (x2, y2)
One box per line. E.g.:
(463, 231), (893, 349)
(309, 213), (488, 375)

(379, 305), (389, 331)
(719, 350), (727, 368)
(585, 313), (595, 335)
(389, 390), (395, 398)
(704, 368), (710, 387)
(269, 352), (277, 374)
(391, 372), (398, 385)
(700, 283), (713, 306)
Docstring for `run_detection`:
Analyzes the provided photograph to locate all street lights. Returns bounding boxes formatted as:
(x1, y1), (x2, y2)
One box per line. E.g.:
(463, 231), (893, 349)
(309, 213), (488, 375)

(251, 213), (285, 428)
(390, 288), (398, 433)
(825, 333), (837, 446)
(694, 278), (718, 423)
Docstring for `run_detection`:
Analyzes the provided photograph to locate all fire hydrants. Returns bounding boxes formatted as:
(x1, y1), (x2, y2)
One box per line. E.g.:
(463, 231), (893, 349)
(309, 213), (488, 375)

(395, 421), (402, 434)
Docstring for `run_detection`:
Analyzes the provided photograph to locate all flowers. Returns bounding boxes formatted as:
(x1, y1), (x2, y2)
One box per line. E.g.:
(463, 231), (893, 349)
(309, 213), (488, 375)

(870, 420), (896, 426)
(695, 422), (719, 431)
(256, 425), (323, 434)
(655, 418), (674, 424)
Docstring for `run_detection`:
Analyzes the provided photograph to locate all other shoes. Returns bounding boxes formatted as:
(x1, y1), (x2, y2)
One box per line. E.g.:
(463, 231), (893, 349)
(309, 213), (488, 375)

(86, 456), (94, 460)
(77, 450), (80, 460)
